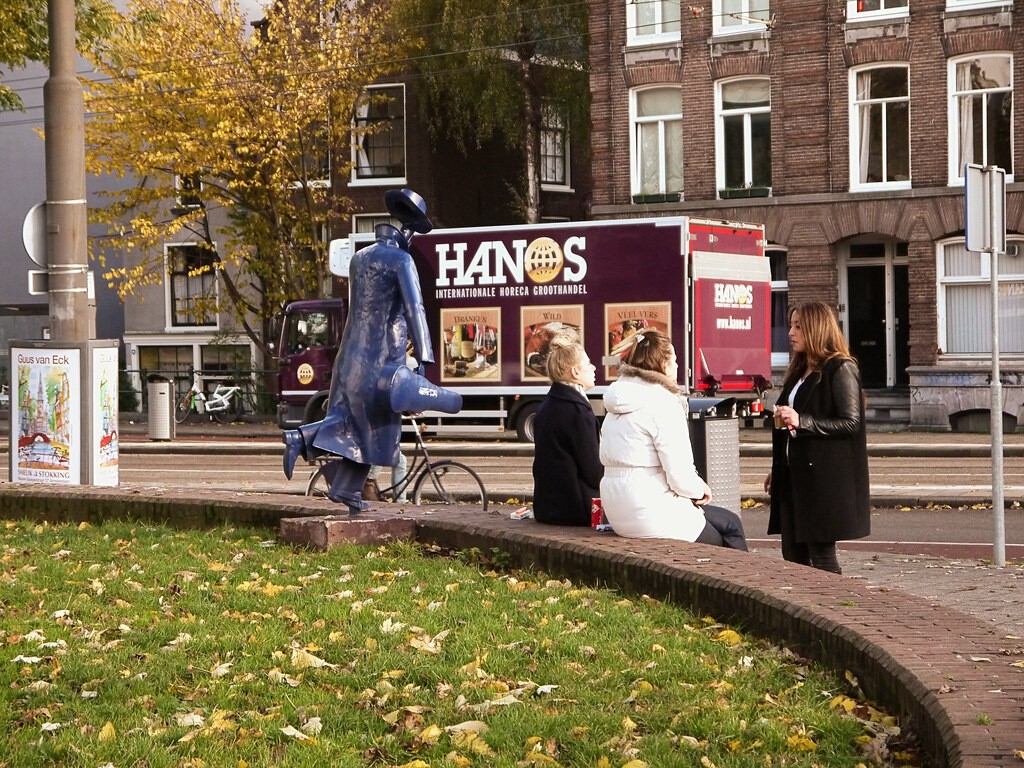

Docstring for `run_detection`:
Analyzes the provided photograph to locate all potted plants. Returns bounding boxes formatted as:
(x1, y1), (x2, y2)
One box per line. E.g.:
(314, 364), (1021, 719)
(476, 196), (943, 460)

(718, 182), (771, 199)
(631, 192), (683, 203)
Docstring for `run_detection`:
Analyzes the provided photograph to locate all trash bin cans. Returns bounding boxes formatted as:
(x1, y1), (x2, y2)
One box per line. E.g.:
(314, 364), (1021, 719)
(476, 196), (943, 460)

(687, 396), (742, 520)
(147, 373), (176, 443)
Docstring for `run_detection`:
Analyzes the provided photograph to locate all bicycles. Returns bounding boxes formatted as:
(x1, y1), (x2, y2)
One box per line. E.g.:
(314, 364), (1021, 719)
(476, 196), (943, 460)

(304, 410), (488, 513)
(174, 364), (242, 424)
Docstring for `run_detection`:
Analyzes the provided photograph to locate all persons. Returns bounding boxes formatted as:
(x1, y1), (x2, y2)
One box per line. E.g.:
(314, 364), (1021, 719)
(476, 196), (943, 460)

(533, 335), (604, 524)
(361, 414), (421, 504)
(598, 330), (749, 552)
(763, 300), (871, 576)
(281, 222), (436, 510)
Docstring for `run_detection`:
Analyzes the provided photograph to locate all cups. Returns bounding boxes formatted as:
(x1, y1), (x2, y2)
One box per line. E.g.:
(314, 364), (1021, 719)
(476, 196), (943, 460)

(591, 498), (604, 528)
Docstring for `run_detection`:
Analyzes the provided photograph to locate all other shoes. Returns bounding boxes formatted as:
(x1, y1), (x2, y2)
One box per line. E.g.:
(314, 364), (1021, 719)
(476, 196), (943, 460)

(363, 482), (378, 502)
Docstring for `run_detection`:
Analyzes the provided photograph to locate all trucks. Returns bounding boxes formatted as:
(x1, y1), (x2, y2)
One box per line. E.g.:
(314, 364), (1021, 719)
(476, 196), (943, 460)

(275, 208), (773, 454)
